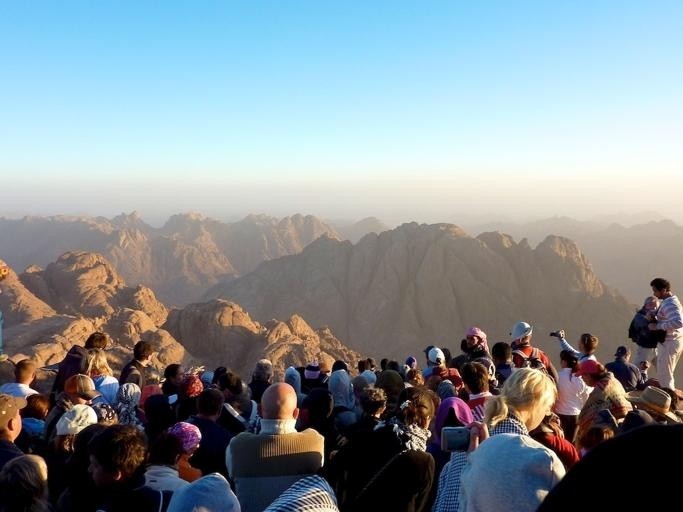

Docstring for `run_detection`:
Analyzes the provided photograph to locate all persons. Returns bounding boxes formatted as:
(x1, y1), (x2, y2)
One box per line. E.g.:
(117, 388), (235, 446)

(625, 295), (676, 384)
(647, 277), (683, 392)
(1, 322), (682, 512)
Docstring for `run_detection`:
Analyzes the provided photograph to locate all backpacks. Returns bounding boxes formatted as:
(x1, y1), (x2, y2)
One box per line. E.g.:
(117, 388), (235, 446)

(511, 348), (548, 373)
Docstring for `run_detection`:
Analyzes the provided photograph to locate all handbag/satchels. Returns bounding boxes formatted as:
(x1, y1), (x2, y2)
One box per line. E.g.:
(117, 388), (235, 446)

(634, 312), (666, 343)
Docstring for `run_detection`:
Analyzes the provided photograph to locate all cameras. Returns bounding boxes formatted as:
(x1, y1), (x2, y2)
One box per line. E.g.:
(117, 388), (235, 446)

(440, 427), (480, 452)
(550, 329), (565, 338)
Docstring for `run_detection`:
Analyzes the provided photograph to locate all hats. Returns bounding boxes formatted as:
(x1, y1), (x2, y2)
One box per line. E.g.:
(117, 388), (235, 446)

(63, 373), (101, 401)
(430, 322), (681, 428)
(319, 363), (329, 373)
(0, 394), (27, 426)
(304, 363), (320, 380)
